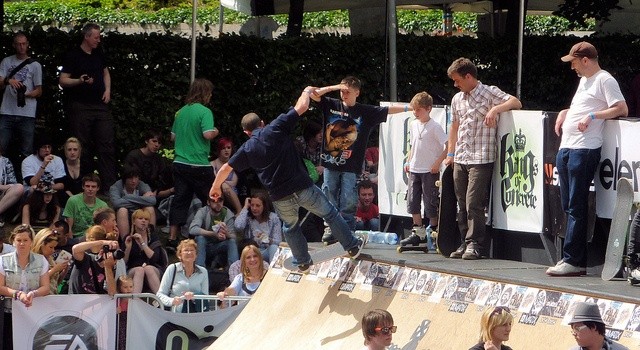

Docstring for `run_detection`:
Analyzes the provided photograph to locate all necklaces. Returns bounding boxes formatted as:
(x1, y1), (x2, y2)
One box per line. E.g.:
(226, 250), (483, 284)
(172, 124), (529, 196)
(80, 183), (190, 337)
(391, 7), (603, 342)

(415, 117), (432, 142)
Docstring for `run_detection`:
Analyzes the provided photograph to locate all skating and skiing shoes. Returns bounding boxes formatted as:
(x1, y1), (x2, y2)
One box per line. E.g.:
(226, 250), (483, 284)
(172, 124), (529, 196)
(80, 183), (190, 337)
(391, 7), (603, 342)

(397, 224), (428, 252)
(426, 224), (439, 254)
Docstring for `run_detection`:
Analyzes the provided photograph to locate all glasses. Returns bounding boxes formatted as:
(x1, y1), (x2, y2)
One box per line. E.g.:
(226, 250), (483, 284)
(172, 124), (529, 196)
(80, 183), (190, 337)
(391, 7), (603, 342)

(374, 326), (396, 333)
(569, 325), (585, 333)
(490, 306), (509, 316)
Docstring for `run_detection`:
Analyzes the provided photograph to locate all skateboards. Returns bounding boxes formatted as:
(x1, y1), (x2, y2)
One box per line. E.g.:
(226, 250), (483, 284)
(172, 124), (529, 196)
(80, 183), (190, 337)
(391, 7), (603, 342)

(601, 177), (635, 281)
(283, 233), (370, 272)
(435, 160), (460, 257)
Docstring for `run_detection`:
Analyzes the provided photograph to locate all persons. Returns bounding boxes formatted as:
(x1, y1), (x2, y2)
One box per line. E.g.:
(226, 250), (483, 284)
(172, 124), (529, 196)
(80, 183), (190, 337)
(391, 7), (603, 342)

(546, 41), (630, 278)
(565, 300), (631, 350)
(353, 178), (379, 232)
(124, 126), (170, 186)
(443, 56), (523, 260)
(0, 29), (44, 160)
(71, 224), (119, 297)
(188, 190), (240, 267)
(21, 142), (66, 226)
(0, 224), (50, 350)
(232, 191), (283, 265)
(296, 118), (324, 168)
(167, 77), (222, 247)
(154, 238), (210, 315)
(61, 135), (91, 206)
(308, 75), (414, 246)
(58, 22), (118, 190)
(398, 91), (449, 247)
(0, 153), (25, 218)
(465, 305), (518, 350)
(62, 171), (110, 239)
(120, 208), (164, 296)
(111, 275), (139, 316)
(207, 85), (367, 275)
(360, 308), (398, 350)
(32, 227), (66, 291)
(108, 162), (157, 239)
(209, 135), (244, 218)
(215, 244), (268, 307)
(227, 238), (271, 306)
(21, 173), (60, 228)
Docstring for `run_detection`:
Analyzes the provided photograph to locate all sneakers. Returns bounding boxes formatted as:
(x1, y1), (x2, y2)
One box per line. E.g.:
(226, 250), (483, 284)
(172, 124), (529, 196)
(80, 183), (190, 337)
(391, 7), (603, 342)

(298, 265), (310, 273)
(321, 227), (332, 241)
(449, 243), (465, 257)
(630, 269), (639, 278)
(461, 242), (486, 259)
(545, 262), (579, 276)
(555, 261), (585, 275)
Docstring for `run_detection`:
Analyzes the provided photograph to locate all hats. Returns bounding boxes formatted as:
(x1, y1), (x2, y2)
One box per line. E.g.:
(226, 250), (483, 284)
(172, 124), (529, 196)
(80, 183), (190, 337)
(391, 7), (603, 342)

(567, 302), (604, 325)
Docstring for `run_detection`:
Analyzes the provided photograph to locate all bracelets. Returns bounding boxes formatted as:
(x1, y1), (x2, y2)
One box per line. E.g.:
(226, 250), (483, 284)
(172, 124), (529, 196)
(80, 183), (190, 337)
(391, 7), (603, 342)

(302, 88), (312, 95)
(588, 111), (597, 121)
(31, 288), (39, 299)
(139, 240), (146, 246)
(13, 288), (23, 302)
(403, 104), (408, 114)
(445, 151), (456, 158)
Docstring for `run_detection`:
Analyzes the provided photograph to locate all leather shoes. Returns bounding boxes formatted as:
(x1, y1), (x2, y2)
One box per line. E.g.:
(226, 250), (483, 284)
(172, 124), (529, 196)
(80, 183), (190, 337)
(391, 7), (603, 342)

(348, 234), (365, 259)
